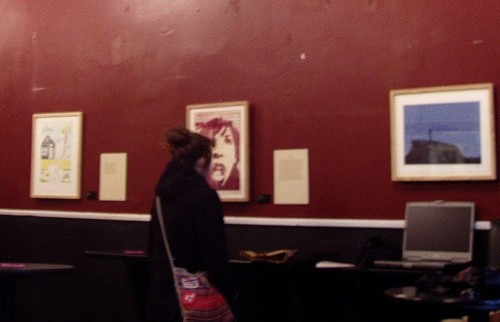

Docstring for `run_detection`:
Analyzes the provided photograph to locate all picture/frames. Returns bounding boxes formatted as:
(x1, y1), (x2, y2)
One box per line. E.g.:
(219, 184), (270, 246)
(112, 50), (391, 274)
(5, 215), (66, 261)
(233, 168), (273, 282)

(183, 100), (252, 202)
(387, 82), (498, 182)
(29, 111), (84, 200)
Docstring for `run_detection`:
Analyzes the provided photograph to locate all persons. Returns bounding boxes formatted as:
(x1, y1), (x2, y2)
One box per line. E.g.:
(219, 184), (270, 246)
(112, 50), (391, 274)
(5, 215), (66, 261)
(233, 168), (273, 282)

(194, 118), (241, 189)
(147, 128), (241, 322)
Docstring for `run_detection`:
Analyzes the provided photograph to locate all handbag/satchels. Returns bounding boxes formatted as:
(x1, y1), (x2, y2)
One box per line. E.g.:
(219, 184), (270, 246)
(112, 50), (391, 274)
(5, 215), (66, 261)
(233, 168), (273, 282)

(174, 266), (234, 322)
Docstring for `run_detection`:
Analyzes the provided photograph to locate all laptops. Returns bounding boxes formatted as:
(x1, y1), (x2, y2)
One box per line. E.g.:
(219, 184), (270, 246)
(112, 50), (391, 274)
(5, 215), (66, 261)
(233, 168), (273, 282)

(373, 200), (475, 269)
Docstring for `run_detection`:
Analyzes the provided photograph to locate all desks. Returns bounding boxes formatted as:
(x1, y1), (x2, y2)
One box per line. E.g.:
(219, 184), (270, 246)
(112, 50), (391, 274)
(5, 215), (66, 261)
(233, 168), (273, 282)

(0, 235), (488, 322)
